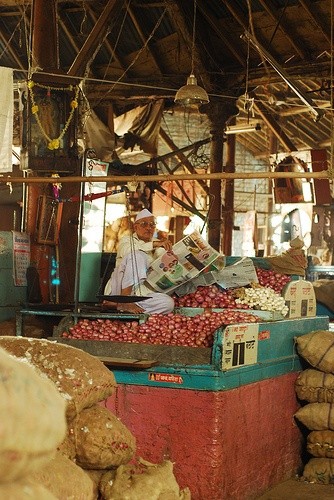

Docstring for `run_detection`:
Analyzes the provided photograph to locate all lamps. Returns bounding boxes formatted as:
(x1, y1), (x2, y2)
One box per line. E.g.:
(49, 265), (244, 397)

(173, 0), (211, 105)
(223, 25), (261, 134)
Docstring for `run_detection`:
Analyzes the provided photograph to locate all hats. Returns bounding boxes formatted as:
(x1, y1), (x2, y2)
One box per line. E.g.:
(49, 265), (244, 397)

(134, 209), (154, 223)
(135, 221), (158, 227)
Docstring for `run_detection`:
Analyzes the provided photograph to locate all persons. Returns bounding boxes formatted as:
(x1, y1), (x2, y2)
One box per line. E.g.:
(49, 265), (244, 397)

(103, 208), (175, 315)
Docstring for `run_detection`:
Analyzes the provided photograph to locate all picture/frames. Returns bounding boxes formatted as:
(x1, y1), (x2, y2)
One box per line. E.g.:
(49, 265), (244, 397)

(37, 195), (64, 245)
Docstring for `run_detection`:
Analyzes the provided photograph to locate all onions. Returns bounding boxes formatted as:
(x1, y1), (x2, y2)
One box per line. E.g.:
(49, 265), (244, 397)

(61, 269), (292, 348)
